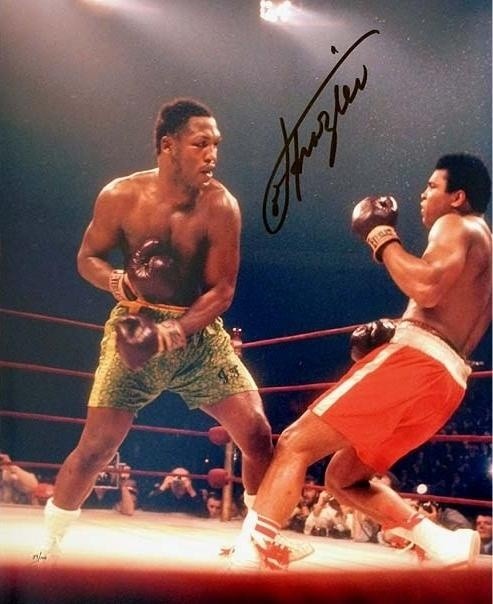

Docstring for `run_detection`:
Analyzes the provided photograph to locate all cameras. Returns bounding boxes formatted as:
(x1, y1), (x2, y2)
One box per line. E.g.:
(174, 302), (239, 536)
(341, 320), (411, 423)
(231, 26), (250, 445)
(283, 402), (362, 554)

(172, 478), (185, 489)
(97, 472), (112, 484)
(422, 501), (440, 513)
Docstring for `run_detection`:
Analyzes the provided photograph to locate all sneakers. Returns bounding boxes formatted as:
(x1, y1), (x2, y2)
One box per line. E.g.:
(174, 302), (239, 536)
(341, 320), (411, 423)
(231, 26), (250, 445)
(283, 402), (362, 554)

(435, 525), (482, 569)
(15, 535), (63, 567)
(271, 532), (316, 563)
(213, 507), (292, 575)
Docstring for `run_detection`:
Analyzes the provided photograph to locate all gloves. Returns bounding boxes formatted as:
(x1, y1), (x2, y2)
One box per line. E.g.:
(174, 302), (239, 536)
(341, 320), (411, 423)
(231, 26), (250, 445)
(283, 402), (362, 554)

(346, 316), (402, 364)
(106, 237), (181, 307)
(348, 192), (398, 265)
(112, 314), (189, 370)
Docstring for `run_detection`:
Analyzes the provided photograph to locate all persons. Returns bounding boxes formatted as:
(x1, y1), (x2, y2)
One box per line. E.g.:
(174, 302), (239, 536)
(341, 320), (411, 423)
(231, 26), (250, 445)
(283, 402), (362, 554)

(212, 149), (493, 568)
(42, 98), (316, 568)
(1, 445), (493, 556)
(380, 370), (492, 444)
